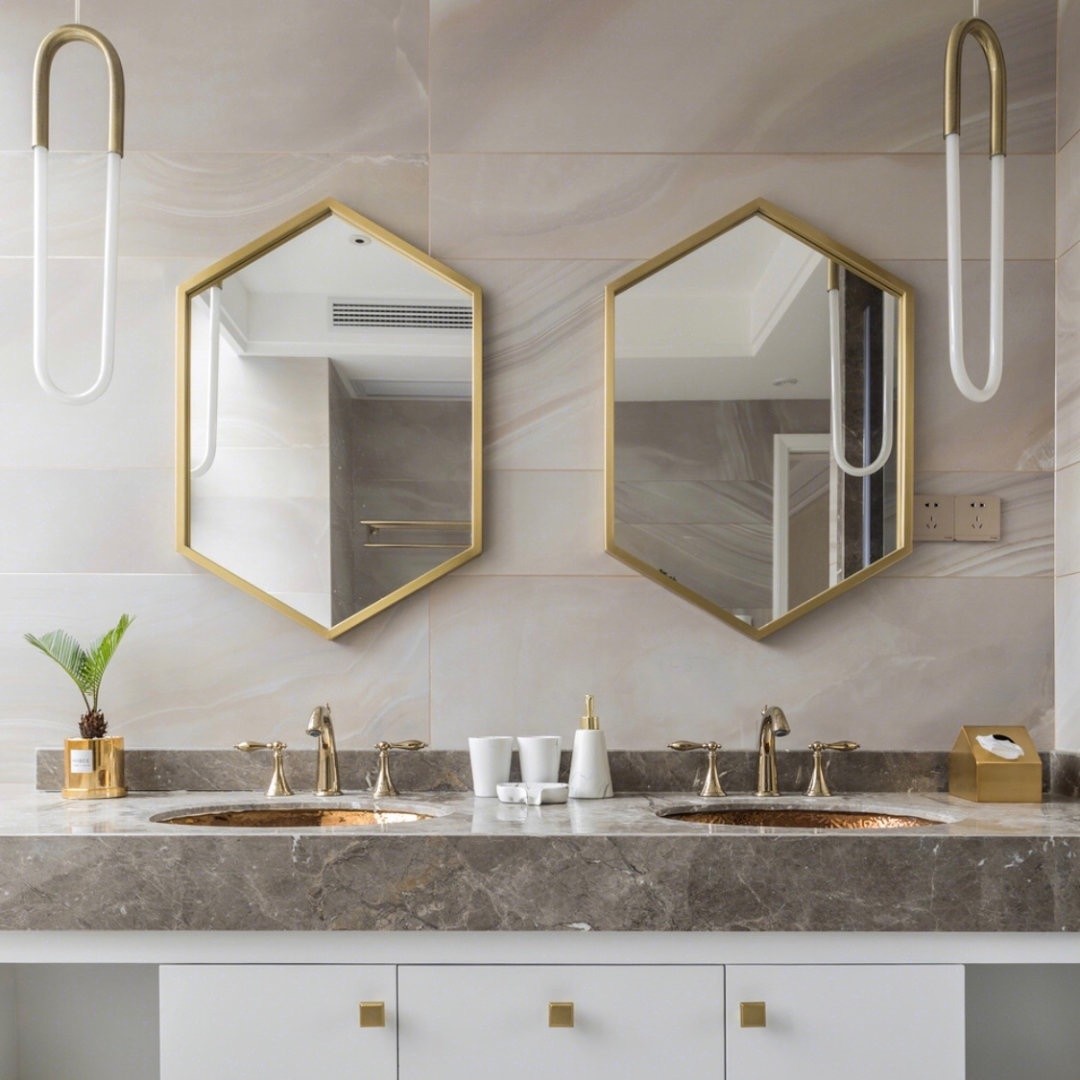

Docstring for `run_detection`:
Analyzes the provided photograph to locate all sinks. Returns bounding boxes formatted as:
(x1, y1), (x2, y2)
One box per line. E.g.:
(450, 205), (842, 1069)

(146, 801), (457, 828)
(653, 798), (963, 832)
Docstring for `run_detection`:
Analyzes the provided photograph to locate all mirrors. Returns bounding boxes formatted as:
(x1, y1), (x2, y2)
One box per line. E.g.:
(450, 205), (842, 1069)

(171, 196), (489, 645)
(602, 197), (915, 646)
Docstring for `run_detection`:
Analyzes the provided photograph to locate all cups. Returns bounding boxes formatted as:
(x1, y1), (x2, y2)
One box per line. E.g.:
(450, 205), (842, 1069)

(469, 736), (513, 798)
(519, 736), (562, 784)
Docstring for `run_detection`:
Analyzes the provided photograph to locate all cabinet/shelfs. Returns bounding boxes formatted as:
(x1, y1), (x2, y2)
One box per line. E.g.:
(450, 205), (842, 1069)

(159, 963), (396, 1080)
(397, 962), (726, 1080)
(725, 963), (965, 1080)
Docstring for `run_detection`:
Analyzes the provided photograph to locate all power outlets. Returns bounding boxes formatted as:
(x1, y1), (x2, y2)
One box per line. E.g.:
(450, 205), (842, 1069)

(913, 492), (954, 543)
(954, 496), (1002, 543)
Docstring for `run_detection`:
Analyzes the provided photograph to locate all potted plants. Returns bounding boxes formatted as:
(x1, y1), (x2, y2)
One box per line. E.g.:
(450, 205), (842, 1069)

(20, 614), (133, 801)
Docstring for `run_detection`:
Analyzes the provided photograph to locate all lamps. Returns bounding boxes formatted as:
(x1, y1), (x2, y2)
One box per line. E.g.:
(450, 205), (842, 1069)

(825, 256), (896, 477)
(943, 0), (1005, 401)
(27, 0), (126, 402)
(190, 281), (223, 479)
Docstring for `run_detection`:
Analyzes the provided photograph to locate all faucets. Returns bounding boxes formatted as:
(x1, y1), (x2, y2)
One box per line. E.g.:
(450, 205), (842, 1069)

(754, 706), (792, 798)
(305, 706), (344, 797)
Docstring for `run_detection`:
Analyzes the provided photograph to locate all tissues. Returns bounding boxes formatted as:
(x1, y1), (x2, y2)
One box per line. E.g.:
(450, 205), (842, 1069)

(947, 724), (1045, 803)
